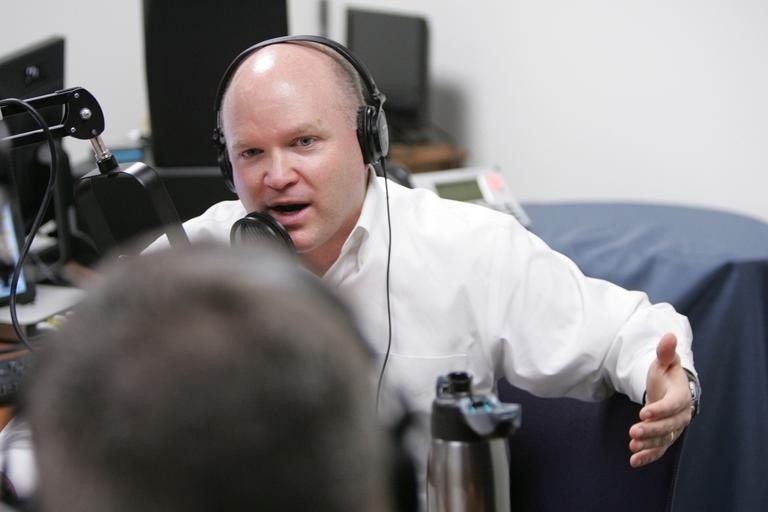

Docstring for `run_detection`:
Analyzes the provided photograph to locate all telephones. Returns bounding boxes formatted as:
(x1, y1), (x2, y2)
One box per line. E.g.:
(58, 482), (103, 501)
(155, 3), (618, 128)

(406, 163), (531, 240)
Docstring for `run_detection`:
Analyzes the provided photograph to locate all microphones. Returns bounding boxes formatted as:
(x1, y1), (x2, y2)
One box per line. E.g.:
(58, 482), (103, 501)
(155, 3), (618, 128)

(72, 154), (189, 263)
(229, 211), (298, 257)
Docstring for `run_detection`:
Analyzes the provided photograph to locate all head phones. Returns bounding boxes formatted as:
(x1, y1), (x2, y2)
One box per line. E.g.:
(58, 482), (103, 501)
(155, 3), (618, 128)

(212, 34), (390, 194)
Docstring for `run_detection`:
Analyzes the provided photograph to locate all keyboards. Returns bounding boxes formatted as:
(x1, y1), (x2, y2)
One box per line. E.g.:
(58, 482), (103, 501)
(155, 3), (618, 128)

(0, 350), (40, 406)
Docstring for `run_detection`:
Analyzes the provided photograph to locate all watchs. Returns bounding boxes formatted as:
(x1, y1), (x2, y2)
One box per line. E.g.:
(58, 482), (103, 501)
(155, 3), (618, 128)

(688, 379), (698, 417)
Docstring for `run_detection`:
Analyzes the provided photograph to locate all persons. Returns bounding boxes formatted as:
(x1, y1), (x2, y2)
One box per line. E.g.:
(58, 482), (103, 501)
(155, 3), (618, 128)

(24, 232), (392, 510)
(1, 37), (700, 510)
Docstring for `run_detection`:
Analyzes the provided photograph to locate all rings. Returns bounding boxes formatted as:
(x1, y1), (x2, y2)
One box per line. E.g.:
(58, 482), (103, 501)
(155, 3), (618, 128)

(665, 431), (674, 445)
